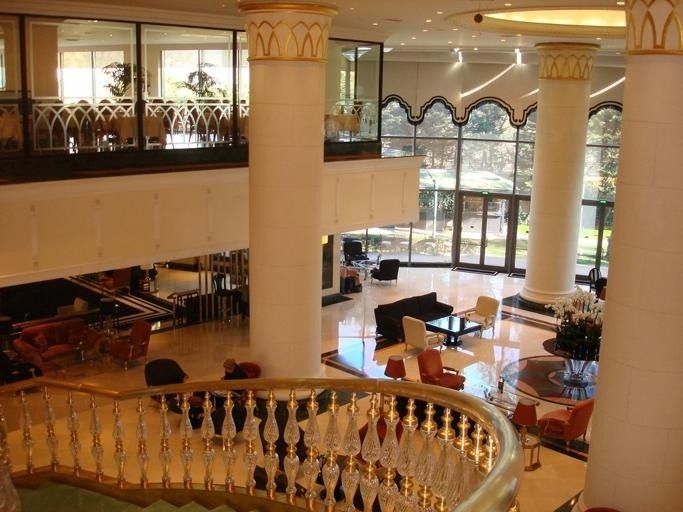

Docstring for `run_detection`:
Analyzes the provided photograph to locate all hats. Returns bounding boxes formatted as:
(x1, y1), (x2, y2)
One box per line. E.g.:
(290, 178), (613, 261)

(223, 358), (236, 370)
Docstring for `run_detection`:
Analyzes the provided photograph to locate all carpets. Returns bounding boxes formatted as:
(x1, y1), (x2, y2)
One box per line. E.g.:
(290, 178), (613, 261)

(0, 276), (190, 375)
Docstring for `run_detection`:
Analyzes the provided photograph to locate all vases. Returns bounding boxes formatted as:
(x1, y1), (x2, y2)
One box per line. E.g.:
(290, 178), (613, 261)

(556, 324), (601, 356)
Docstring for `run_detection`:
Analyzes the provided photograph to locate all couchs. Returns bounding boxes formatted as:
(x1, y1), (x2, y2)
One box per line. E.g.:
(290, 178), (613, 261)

(340, 266), (363, 293)
(13, 318), (106, 369)
(374, 292), (453, 340)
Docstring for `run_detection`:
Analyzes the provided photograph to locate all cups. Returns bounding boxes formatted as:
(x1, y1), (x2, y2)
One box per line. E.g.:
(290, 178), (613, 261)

(449, 316), (454, 330)
(460, 317), (465, 331)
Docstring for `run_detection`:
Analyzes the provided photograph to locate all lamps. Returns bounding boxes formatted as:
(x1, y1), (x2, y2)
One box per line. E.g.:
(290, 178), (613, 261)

(384, 355), (406, 380)
(511, 398), (537, 442)
(140, 264), (154, 281)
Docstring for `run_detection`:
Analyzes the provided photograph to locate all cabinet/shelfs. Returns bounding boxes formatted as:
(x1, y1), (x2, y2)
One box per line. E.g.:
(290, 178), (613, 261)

(517, 432), (541, 471)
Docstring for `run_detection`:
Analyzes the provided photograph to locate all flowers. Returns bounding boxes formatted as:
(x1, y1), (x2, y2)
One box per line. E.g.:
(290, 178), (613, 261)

(543, 283), (606, 332)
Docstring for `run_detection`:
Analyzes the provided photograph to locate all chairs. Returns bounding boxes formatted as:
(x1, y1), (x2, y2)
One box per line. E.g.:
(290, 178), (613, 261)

(588, 268), (603, 294)
(338, 101), (363, 141)
(186, 99), (249, 146)
(418, 349), (465, 391)
(144, 358), (213, 419)
(218, 362), (262, 415)
(370, 259), (400, 287)
(464, 296), (500, 339)
(50, 98), (182, 152)
(344, 242), (369, 272)
(535, 398), (594, 453)
(402, 316), (445, 355)
(0, 350), (43, 394)
(108, 319), (151, 371)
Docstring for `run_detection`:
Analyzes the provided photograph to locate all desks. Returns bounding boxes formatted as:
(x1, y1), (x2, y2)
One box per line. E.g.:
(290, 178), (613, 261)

(460, 381), (539, 419)
(595, 282), (607, 300)
(424, 315), (482, 346)
(351, 260), (378, 280)
(543, 337), (600, 401)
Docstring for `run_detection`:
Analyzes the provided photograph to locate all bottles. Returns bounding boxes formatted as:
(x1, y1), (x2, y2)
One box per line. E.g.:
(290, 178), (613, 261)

(498, 376), (504, 394)
